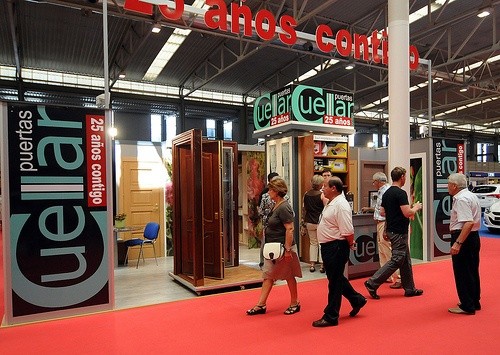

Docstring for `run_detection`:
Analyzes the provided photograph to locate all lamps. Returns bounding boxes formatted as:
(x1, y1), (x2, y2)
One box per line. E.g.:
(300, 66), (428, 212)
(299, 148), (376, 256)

(151, 21), (162, 34)
(96, 92), (111, 108)
(476, 7), (494, 18)
(459, 86), (470, 93)
(344, 63), (355, 70)
(419, 125), (429, 138)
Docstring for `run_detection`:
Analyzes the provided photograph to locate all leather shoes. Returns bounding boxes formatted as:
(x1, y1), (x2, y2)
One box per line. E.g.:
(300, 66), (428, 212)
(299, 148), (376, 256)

(404, 288), (423, 296)
(390, 282), (403, 288)
(457, 301), (481, 309)
(312, 318), (338, 327)
(364, 281), (380, 299)
(448, 306), (475, 314)
(350, 298), (367, 317)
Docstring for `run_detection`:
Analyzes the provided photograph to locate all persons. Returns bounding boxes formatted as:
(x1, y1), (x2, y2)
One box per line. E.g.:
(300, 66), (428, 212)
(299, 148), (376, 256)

(448, 173), (481, 315)
(312, 176), (368, 328)
(246, 176), (300, 315)
(256, 172), (295, 271)
(319, 169), (346, 201)
(302, 174), (329, 273)
(361, 172), (404, 289)
(363, 166), (424, 300)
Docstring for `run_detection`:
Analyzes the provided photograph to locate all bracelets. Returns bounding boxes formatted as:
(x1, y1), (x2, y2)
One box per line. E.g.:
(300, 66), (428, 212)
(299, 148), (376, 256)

(455, 241), (463, 244)
(285, 250), (292, 253)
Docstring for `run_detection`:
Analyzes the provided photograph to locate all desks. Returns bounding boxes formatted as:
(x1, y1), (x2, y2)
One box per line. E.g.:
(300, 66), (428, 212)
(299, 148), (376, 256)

(342, 212), (381, 280)
(113, 226), (138, 267)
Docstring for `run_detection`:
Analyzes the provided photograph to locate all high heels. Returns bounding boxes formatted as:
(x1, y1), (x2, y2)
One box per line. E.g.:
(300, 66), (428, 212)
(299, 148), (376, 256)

(247, 304), (266, 315)
(284, 302), (300, 314)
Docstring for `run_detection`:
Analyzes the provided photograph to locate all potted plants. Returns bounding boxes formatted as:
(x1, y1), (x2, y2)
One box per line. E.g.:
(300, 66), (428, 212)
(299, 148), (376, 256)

(115, 213), (127, 229)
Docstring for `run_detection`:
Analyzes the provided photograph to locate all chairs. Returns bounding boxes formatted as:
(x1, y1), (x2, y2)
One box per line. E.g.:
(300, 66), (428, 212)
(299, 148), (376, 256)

(122, 222), (160, 270)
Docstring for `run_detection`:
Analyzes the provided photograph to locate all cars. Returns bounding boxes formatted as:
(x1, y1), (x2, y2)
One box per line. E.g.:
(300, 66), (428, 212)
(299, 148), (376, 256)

(470, 183), (500, 212)
(482, 199), (500, 235)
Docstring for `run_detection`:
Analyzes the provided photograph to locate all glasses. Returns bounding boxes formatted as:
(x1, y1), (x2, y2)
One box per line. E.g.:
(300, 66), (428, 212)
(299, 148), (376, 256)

(372, 180), (377, 186)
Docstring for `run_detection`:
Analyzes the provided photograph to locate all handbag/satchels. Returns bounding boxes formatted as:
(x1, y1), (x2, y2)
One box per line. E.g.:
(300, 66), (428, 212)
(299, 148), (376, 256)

(300, 222), (308, 237)
(263, 242), (286, 264)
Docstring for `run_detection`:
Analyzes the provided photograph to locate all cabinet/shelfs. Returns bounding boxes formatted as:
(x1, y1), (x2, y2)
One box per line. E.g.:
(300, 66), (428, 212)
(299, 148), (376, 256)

(313, 132), (350, 196)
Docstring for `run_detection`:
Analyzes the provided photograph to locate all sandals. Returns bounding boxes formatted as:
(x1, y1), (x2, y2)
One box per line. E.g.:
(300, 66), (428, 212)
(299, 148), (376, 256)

(310, 266), (315, 272)
(320, 267), (325, 273)
